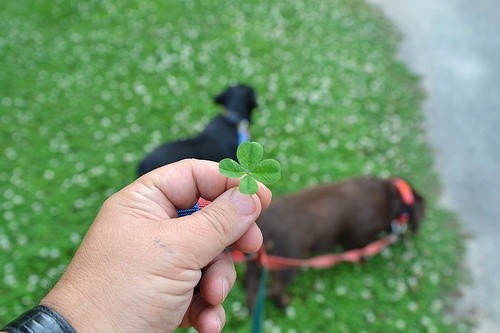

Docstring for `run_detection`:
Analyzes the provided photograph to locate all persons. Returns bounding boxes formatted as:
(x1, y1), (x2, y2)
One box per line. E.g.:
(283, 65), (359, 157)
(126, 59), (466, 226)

(1, 156), (272, 333)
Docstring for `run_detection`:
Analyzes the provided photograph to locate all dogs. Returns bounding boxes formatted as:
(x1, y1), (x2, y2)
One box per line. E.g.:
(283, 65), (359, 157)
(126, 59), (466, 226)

(244, 175), (424, 315)
(138, 84), (259, 178)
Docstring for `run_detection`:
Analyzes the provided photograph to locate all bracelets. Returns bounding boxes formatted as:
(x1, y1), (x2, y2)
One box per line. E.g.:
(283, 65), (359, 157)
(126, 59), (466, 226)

(2, 305), (74, 332)
